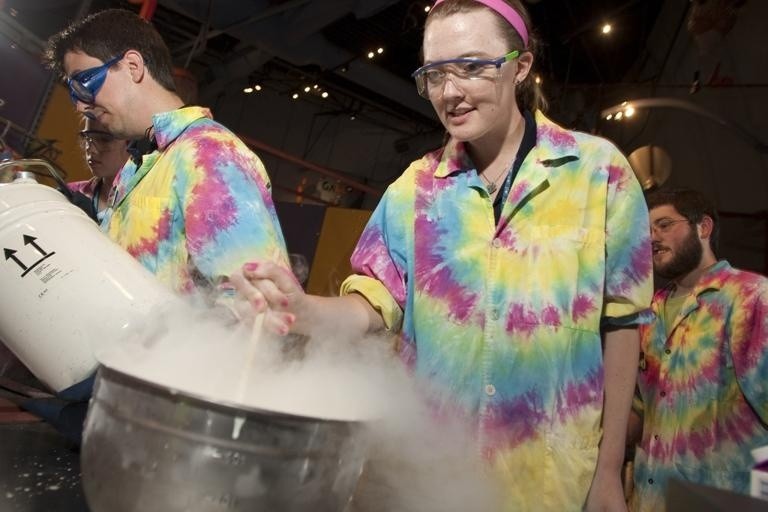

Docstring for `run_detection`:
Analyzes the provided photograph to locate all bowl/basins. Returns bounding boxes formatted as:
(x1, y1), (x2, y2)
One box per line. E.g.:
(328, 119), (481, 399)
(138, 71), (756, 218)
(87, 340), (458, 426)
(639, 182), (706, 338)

(79, 357), (382, 512)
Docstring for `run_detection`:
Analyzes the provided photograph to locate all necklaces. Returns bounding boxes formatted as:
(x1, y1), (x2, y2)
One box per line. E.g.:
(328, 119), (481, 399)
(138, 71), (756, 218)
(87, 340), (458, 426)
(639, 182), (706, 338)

(462, 151), (519, 198)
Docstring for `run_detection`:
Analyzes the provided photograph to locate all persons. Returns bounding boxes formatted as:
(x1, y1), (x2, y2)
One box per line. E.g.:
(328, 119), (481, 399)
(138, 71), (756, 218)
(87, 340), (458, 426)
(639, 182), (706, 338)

(42, 6), (300, 356)
(57, 113), (133, 222)
(225, 2), (655, 510)
(628, 183), (768, 510)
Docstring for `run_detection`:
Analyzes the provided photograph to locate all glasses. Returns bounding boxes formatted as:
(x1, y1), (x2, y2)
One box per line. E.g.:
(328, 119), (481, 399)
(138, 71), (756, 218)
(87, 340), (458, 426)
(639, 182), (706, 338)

(77, 130), (126, 152)
(411, 50), (519, 101)
(66, 57), (120, 104)
(650, 219), (689, 232)
